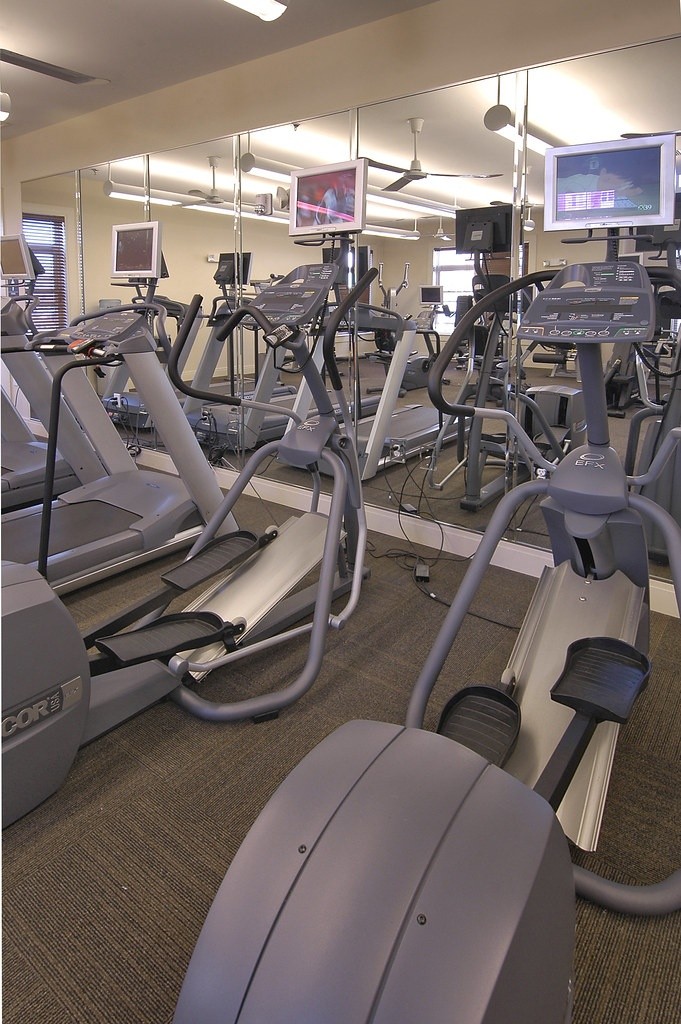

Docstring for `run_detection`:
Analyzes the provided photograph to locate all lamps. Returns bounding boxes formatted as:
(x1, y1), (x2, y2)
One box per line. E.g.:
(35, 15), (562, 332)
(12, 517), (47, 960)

(366, 183), (465, 219)
(222, 0), (286, 22)
(103, 163), (289, 224)
(484, 75), (567, 158)
(521, 208), (535, 231)
(360, 219), (421, 241)
(240, 133), (304, 183)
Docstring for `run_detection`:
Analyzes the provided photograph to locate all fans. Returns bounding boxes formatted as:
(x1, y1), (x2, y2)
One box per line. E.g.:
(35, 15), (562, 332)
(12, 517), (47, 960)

(490, 165), (544, 207)
(358, 118), (504, 191)
(172, 156), (258, 207)
(0, 48), (111, 90)
(400, 216), (455, 240)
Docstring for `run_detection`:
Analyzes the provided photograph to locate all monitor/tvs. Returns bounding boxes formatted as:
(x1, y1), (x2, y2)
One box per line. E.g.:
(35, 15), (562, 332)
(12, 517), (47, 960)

(213, 252), (254, 285)
(618, 251), (643, 266)
(0, 234), (45, 280)
(289, 158), (369, 237)
(110, 221), (170, 278)
(544, 134), (676, 232)
(635, 193), (681, 251)
(323, 245), (370, 283)
(419, 285), (443, 305)
(455, 204), (513, 255)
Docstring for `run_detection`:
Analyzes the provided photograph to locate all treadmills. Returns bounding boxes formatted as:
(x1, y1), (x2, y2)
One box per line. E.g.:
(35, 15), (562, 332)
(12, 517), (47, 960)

(178, 251), (381, 449)
(1, 293), (109, 512)
(99, 249), (299, 430)
(282, 304), (471, 485)
(2, 302), (241, 600)
